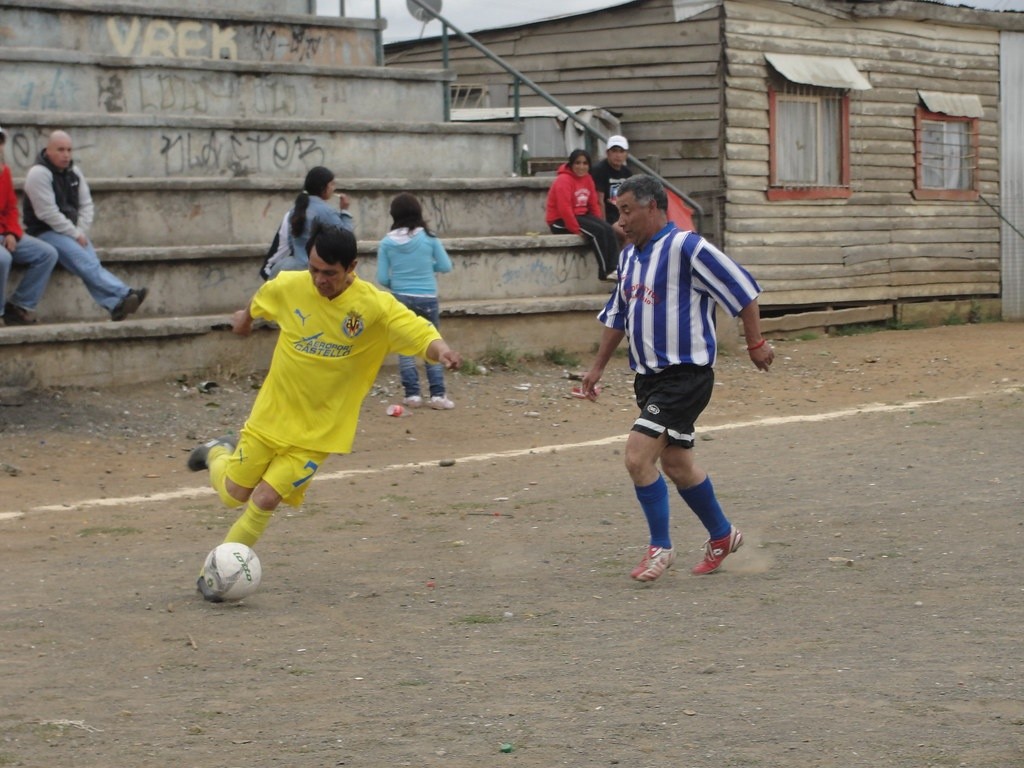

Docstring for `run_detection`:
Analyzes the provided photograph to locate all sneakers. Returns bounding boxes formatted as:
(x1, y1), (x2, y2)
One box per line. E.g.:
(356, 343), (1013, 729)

(632, 545), (678, 582)
(693, 525), (744, 576)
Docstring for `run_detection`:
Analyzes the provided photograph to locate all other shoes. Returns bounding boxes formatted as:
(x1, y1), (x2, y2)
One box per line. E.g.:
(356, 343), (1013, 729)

(190, 434), (238, 472)
(600, 269), (620, 283)
(430, 397), (455, 410)
(403, 395), (423, 407)
(128, 288), (148, 313)
(111, 294), (139, 321)
(5, 304), (36, 324)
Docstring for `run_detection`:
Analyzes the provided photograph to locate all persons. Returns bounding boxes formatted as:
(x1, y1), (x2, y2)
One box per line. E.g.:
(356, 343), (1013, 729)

(1, 128), (58, 326)
(577, 174), (776, 583)
(268, 164), (358, 272)
(184, 220), (461, 544)
(545, 149), (623, 279)
(23, 129), (150, 322)
(377, 190), (458, 408)
(590, 134), (648, 251)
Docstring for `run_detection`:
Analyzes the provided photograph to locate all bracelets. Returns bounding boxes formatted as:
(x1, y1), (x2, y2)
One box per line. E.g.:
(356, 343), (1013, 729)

(746, 339), (766, 350)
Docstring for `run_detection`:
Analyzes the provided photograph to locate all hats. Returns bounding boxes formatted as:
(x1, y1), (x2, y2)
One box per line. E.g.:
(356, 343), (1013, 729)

(606, 134), (630, 152)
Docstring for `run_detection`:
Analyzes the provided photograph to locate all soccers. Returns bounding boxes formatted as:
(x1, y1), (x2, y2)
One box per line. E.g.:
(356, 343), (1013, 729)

(199, 543), (263, 603)
